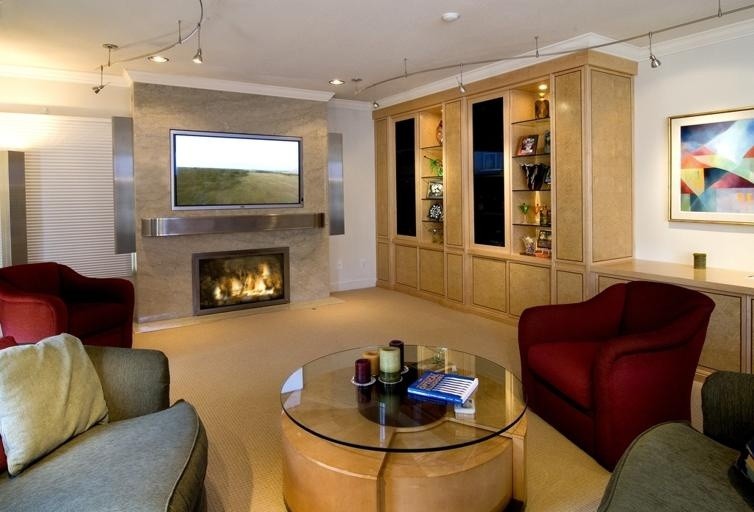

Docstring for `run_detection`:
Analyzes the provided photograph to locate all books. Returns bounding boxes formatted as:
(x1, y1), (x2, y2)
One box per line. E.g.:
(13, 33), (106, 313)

(407, 371), (480, 404)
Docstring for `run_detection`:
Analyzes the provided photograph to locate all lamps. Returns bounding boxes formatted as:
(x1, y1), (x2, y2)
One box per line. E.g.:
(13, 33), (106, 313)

(91, 63), (113, 96)
(192, 22), (204, 64)
(649, 33), (661, 69)
(460, 63), (467, 95)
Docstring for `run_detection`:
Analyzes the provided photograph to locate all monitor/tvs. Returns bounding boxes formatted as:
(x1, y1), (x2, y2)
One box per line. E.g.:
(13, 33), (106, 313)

(171, 129), (304, 211)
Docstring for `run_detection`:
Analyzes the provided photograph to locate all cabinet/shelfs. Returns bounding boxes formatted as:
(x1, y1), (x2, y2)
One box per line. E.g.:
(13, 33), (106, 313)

(370, 46), (640, 329)
(594, 258), (754, 384)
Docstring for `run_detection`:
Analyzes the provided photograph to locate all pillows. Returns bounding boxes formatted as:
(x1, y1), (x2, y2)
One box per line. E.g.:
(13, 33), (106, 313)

(0, 336), (18, 473)
(0, 331), (110, 477)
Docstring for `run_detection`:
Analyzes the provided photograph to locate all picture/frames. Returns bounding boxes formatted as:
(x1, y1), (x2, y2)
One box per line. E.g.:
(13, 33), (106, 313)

(667, 105), (754, 225)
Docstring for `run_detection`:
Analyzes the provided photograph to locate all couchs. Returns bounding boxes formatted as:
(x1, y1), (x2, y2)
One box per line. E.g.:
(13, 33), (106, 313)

(518, 280), (717, 474)
(597, 371), (753, 512)
(1, 262), (135, 348)
(1, 342), (209, 512)
